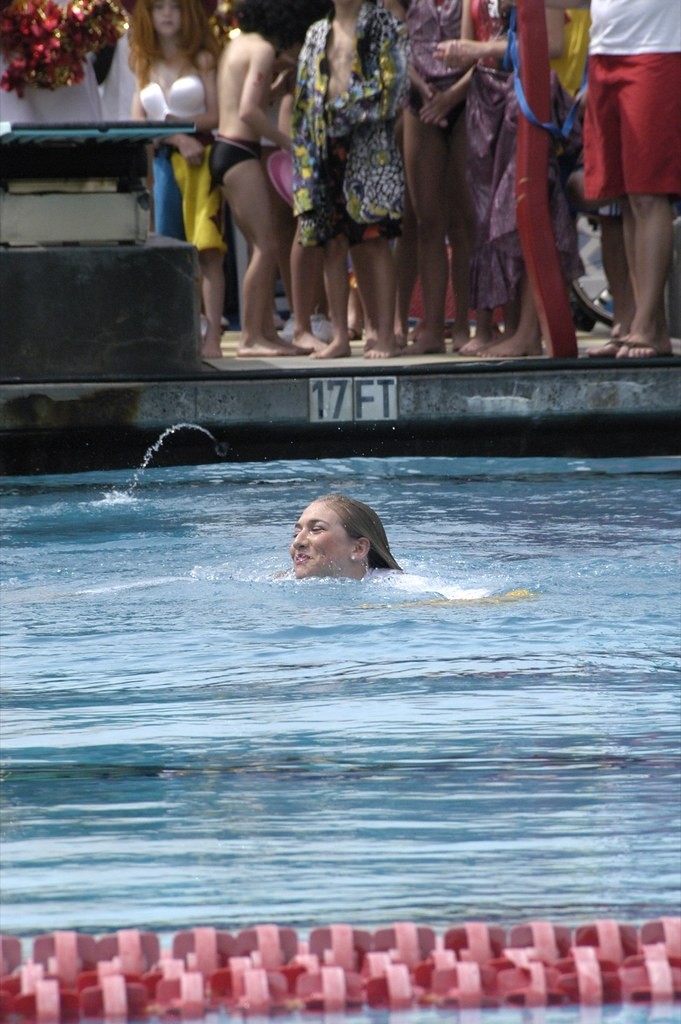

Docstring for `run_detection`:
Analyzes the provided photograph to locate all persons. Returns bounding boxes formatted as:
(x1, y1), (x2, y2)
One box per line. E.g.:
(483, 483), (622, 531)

(288, 495), (403, 582)
(0, 1), (680, 359)
